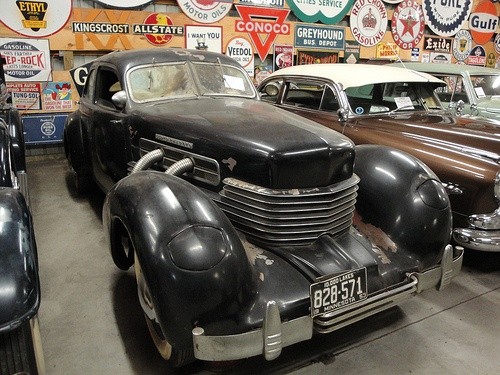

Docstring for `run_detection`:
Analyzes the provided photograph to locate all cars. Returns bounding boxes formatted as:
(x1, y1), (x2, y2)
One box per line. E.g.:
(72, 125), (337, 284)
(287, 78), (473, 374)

(61, 49), (468, 372)
(1, 48), (51, 375)
(254, 62), (500, 258)
(388, 61), (499, 126)
